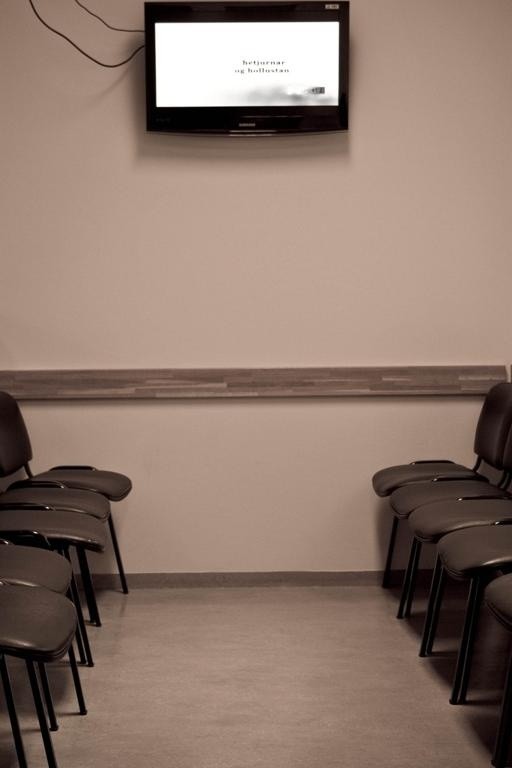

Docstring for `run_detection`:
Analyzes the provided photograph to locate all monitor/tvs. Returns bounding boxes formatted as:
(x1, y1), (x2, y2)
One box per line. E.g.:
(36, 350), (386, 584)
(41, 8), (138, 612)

(143, 0), (350, 139)
